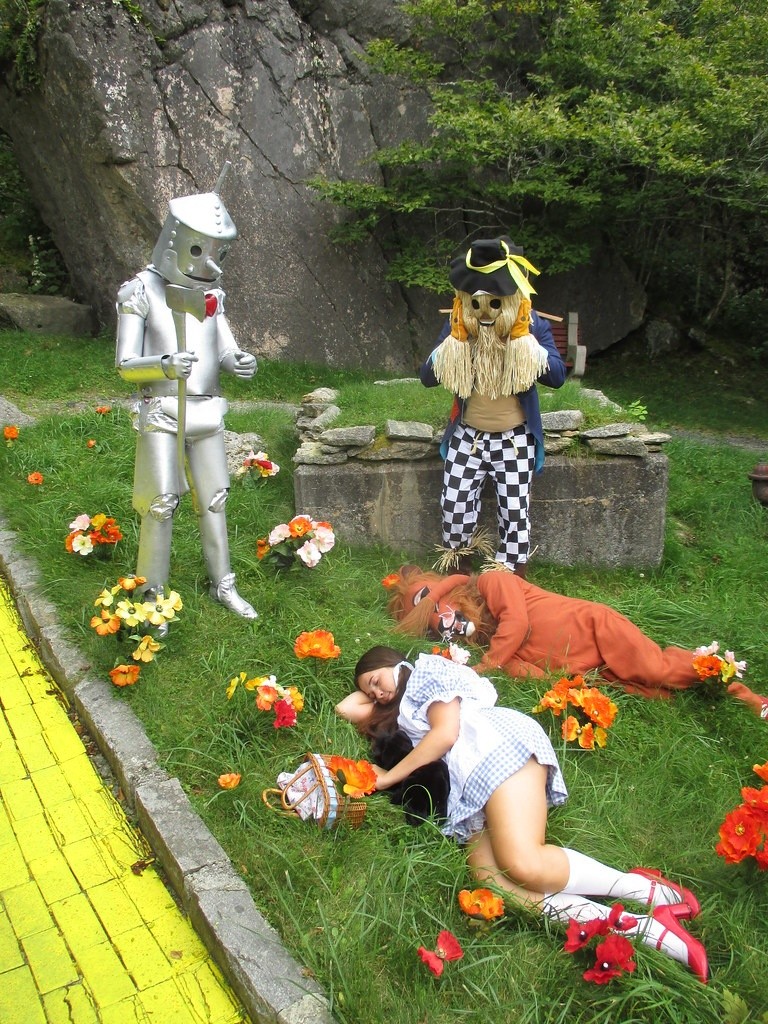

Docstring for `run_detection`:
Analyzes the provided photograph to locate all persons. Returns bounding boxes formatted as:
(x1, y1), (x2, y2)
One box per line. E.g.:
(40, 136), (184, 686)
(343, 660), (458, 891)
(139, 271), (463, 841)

(115, 192), (258, 640)
(420, 225), (567, 581)
(334, 645), (709, 983)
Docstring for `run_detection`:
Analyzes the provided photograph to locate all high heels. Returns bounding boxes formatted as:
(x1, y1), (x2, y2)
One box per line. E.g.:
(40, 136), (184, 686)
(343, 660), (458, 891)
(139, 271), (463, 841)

(651, 903), (707, 983)
(629, 868), (699, 921)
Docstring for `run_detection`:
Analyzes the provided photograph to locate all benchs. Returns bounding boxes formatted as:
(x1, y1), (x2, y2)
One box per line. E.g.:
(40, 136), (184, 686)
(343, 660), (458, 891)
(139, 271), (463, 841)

(543, 312), (587, 378)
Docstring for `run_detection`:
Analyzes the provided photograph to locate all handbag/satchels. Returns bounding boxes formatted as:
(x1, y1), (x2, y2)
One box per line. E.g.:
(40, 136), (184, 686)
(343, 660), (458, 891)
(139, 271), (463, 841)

(262, 750), (366, 829)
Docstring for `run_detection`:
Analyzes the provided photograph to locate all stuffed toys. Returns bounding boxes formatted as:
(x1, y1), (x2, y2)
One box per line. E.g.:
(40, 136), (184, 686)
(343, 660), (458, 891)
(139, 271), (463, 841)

(386, 565), (768, 721)
